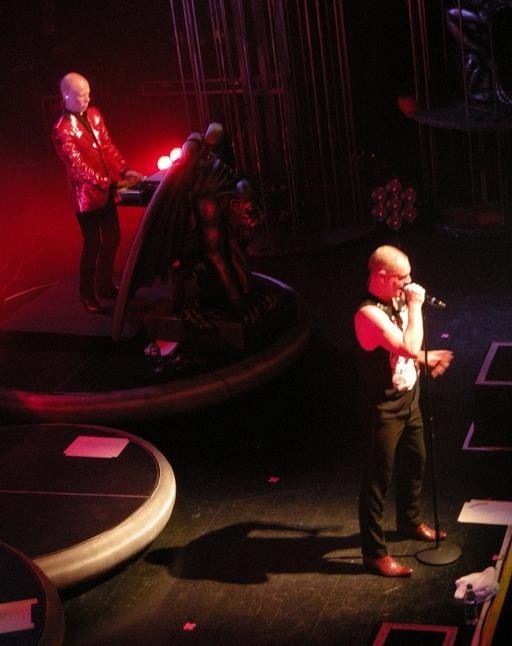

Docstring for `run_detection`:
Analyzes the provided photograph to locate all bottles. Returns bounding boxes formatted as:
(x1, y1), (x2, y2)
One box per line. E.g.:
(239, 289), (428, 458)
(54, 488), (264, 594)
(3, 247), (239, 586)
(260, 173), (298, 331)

(463, 583), (479, 627)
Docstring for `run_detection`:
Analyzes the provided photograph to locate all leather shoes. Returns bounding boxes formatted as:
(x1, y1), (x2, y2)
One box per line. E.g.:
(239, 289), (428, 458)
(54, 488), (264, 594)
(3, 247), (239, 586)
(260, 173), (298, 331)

(414, 521), (448, 543)
(362, 551), (415, 577)
(80, 293), (102, 314)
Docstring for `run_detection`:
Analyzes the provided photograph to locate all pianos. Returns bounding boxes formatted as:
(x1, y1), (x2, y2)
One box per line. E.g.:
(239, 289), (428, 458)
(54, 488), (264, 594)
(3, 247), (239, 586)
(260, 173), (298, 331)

(117, 169), (166, 206)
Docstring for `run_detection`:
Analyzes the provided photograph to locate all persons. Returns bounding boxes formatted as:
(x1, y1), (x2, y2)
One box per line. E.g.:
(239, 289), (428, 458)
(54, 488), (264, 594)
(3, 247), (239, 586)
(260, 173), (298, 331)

(350, 244), (458, 581)
(50, 69), (144, 313)
(201, 119), (227, 145)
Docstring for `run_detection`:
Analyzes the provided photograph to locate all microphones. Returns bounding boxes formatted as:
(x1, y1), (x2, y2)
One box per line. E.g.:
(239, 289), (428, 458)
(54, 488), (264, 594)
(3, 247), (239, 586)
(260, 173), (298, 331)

(403, 284), (448, 312)
